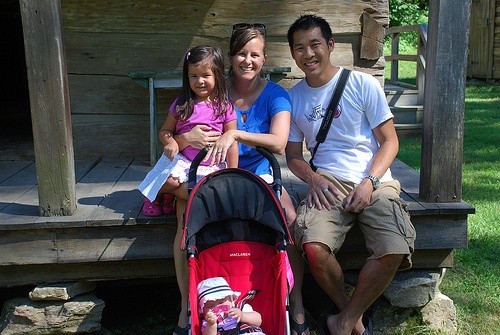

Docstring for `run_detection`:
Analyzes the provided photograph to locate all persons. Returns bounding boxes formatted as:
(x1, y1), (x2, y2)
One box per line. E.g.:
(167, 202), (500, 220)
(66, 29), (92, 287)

(197, 277), (267, 335)
(142, 45), (239, 217)
(173, 23), (311, 335)
(284, 14), (416, 335)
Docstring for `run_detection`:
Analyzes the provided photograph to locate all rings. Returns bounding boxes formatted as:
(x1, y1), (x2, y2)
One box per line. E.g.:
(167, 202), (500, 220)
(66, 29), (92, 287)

(217, 150), (222, 153)
(322, 188), (329, 193)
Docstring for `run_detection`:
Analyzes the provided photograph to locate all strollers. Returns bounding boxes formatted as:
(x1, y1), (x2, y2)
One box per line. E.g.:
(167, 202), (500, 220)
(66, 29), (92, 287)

(180, 145), (294, 335)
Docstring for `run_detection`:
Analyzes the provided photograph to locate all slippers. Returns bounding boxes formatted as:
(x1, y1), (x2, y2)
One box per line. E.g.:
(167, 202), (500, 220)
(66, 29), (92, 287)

(290, 316), (310, 335)
(142, 197), (160, 216)
(160, 194), (176, 213)
(172, 324), (191, 335)
(317, 314), (332, 335)
(361, 316), (373, 335)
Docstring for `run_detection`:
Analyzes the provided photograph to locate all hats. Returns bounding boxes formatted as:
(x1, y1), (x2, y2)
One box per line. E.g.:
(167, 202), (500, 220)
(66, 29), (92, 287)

(197, 277), (241, 313)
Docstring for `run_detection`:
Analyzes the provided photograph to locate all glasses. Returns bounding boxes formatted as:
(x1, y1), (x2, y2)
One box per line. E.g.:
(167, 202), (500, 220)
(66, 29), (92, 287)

(232, 23), (266, 35)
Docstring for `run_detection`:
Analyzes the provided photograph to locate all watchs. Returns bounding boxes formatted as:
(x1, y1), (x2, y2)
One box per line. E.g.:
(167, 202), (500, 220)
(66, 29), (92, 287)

(364, 174), (380, 190)
